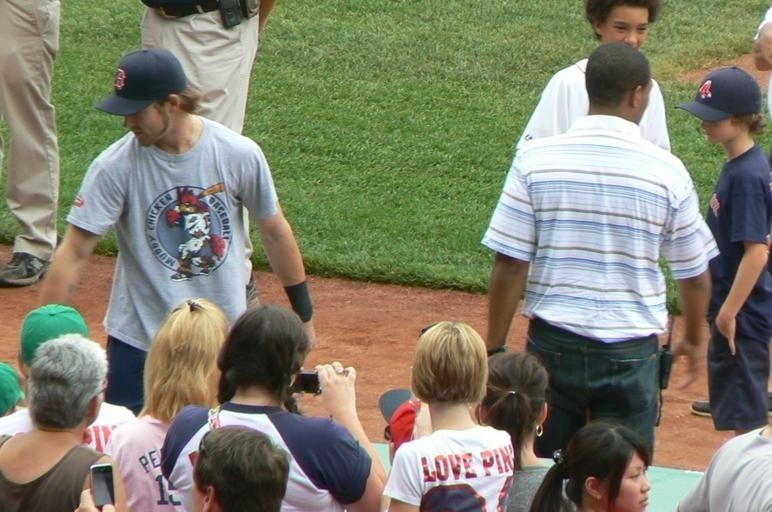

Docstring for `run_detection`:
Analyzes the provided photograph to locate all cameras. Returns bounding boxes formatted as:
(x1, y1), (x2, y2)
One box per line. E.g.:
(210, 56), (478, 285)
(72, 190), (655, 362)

(292, 371), (323, 394)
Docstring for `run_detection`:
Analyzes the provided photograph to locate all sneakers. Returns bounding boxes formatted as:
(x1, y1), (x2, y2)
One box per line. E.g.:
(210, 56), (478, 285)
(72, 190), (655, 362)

(0, 250), (54, 288)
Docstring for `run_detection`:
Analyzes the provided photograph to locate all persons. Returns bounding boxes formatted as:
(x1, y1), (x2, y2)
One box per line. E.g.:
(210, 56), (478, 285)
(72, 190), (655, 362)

(690, 4), (772, 417)
(0, 298), (772, 511)
(35, 47), (316, 418)
(673, 67), (772, 438)
(485, 41), (722, 468)
(0, 0), (62, 285)
(513, 1), (673, 157)
(139, 1), (276, 308)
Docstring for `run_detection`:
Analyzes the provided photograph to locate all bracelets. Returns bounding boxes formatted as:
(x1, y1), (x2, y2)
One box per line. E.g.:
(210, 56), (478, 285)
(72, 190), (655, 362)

(283, 278), (313, 323)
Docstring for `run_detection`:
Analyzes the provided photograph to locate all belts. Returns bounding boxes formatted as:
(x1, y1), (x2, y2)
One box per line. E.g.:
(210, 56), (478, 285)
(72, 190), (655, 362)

(153, 0), (222, 22)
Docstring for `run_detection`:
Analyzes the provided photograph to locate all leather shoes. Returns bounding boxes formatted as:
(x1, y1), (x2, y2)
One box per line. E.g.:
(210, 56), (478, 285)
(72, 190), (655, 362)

(690, 397), (714, 417)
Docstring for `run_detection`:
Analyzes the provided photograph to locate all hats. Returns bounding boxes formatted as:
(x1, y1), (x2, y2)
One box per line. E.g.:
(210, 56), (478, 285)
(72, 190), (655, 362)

(389, 397), (425, 455)
(0, 360), (27, 417)
(673, 64), (765, 124)
(20, 302), (92, 365)
(94, 47), (191, 118)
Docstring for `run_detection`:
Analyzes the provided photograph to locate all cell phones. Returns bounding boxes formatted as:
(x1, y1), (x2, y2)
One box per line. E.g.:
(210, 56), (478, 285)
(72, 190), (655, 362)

(90, 464), (115, 512)
